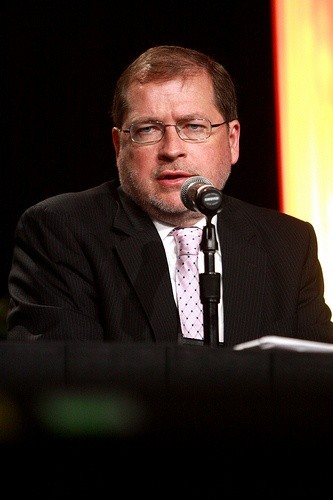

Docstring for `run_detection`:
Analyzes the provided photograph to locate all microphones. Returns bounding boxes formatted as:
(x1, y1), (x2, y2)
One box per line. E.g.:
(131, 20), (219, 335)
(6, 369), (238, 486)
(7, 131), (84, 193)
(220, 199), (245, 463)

(180, 178), (223, 214)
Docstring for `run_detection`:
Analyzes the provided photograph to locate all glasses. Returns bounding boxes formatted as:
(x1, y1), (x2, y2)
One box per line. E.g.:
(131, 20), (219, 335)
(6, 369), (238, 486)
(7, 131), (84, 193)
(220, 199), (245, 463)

(119, 118), (227, 145)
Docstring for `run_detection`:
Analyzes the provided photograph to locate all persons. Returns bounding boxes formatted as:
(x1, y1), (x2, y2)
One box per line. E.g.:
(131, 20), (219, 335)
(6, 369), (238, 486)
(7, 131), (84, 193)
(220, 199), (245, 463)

(4, 46), (333, 343)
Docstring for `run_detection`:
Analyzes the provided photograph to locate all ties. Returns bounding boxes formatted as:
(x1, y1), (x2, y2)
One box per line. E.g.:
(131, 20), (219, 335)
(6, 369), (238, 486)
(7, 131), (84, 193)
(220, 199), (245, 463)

(168, 228), (204, 341)
